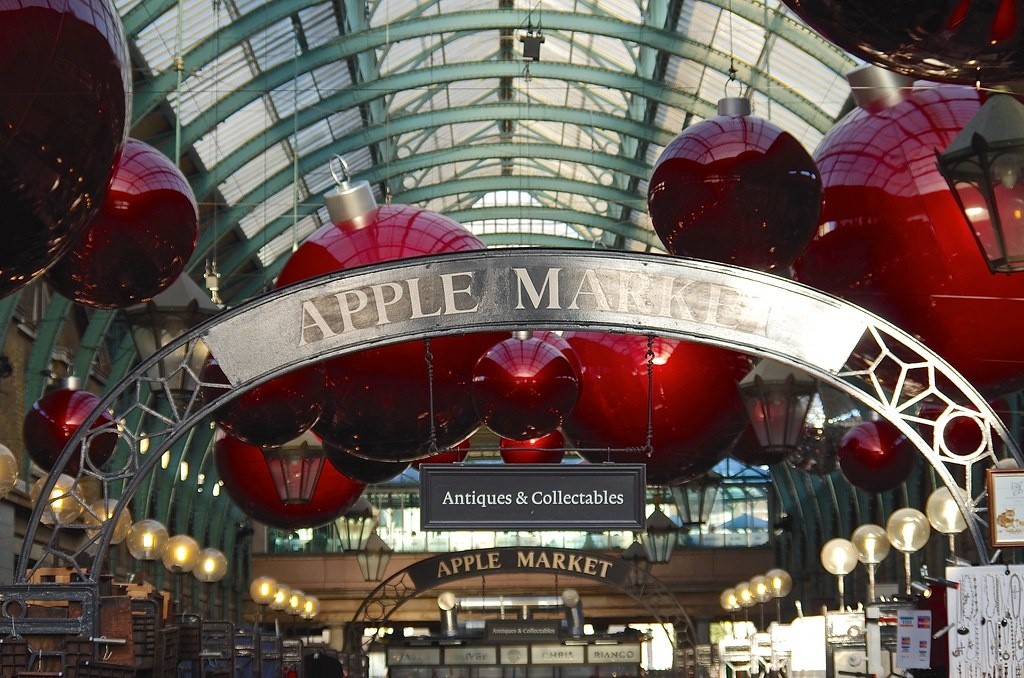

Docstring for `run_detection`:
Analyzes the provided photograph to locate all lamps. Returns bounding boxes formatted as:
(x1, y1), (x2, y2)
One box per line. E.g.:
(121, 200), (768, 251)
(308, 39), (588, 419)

(886, 507), (931, 593)
(32, 473), (85, 567)
(721, 568), (793, 641)
(925, 486), (975, 559)
(162, 533), (199, 613)
(623, 532), (652, 588)
(334, 495), (379, 552)
(0, 443), (18, 500)
(853, 523), (890, 602)
(909, 582), (933, 597)
(127, 1), (226, 405)
(821, 537), (859, 609)
(637, 484), (682, 565)
(193, 547), (227, 615)
(668, 470), (725, 525)
(250, 576), (321, 641)
(260, 429), (329, 504)
(354, 483), (395, 582)
(84, 500), (132, 570)
(124, 520), (170, 583)
(734, 358), (821, 453)
